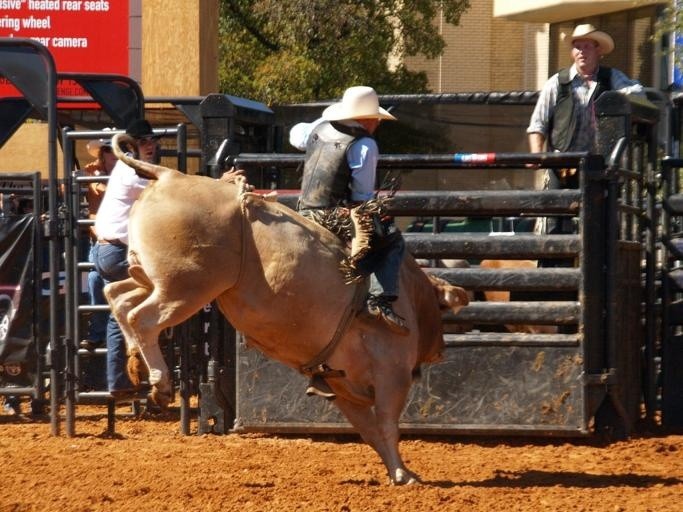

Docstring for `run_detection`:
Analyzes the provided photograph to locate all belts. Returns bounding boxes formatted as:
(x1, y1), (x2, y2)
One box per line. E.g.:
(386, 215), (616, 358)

(98, 239), (122, 245)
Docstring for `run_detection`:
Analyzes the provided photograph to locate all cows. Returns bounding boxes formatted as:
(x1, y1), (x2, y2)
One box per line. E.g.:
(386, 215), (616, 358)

(102, 118), (471, 485)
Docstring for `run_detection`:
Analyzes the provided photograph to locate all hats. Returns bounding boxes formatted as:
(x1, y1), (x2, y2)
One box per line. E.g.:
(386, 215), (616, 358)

(559, 24), (615, 54)
(126, 120), (168, 139)
(87, 126), (120, 158)
(321, 87), (398, 122)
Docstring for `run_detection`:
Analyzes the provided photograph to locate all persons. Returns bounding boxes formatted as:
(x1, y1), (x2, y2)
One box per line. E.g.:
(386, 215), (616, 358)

(73, 127), (128, 361)
(286, 86), (411, 336)
(526, 22), (653, 290)
(87, 121), (247, 403)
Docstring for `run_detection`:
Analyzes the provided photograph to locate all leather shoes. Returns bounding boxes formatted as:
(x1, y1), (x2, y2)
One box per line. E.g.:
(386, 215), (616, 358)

(110, 382), (142, 392)
(79, 339), (97, 349)
(367, 296), (410, 330)
